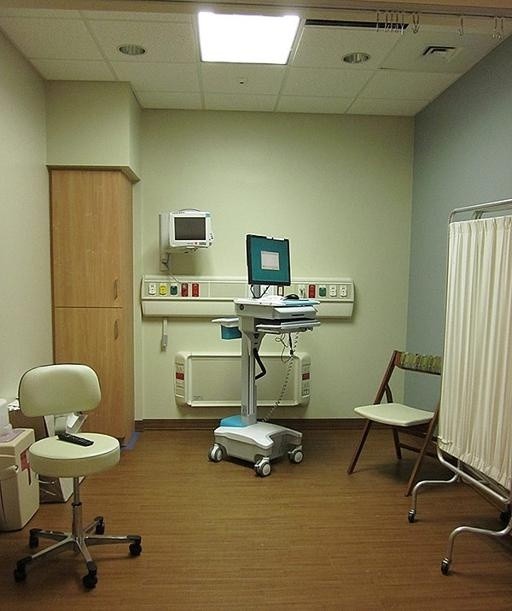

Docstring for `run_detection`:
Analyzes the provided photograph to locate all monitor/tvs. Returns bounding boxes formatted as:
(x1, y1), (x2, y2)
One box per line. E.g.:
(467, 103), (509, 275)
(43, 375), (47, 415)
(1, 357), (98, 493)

(246, 234), (290, 286)
(168, 211), (211, 247)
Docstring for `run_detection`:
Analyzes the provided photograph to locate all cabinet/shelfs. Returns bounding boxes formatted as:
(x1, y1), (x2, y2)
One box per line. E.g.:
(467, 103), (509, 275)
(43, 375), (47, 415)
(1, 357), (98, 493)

(6, 399), (91, 506)
(46, 165), (138, 450)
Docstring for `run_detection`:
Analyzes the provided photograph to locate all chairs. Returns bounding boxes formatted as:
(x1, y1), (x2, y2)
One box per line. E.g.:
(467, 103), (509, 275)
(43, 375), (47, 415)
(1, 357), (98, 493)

(341, 345), (456, 500)
(14, 359), (147, 597)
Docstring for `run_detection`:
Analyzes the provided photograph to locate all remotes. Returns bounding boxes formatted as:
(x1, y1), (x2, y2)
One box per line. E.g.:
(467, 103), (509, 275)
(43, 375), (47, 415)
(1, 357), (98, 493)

(58, 432), (94, 446)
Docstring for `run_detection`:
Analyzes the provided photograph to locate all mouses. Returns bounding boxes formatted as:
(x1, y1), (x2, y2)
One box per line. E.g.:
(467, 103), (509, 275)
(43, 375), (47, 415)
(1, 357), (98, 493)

(286, 294), (299, 299)
